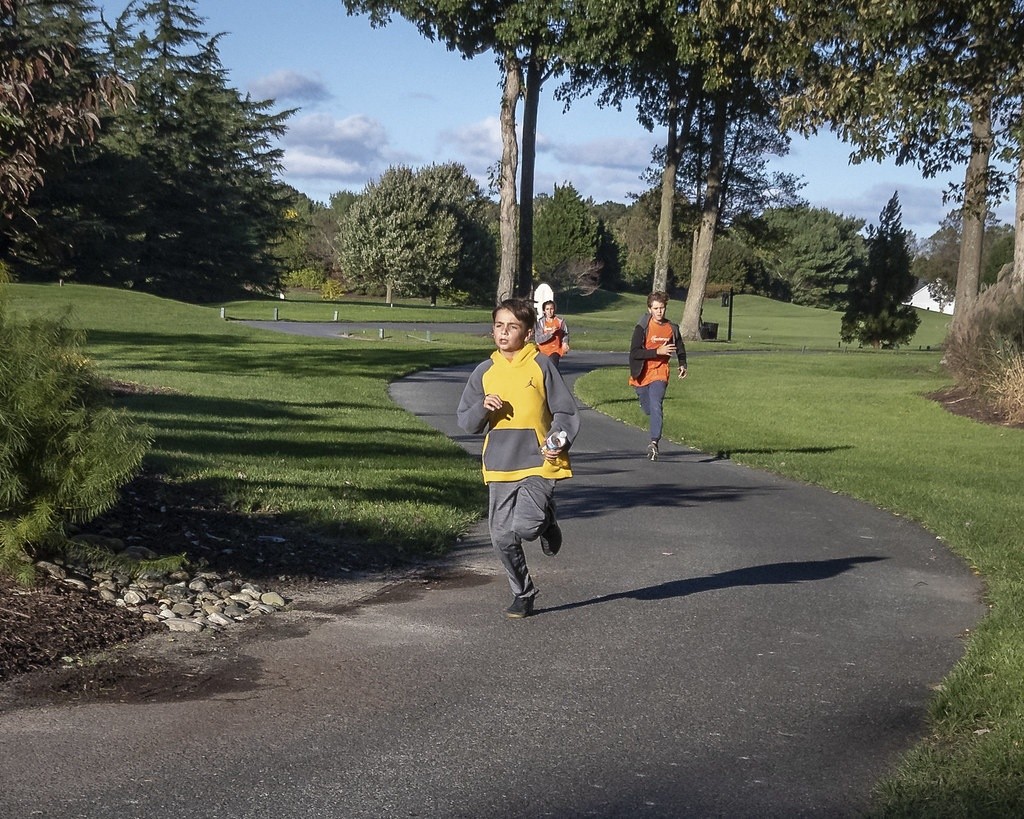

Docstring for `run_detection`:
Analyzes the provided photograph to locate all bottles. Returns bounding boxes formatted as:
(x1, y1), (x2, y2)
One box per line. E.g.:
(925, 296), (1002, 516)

(541, 431), (567, 458)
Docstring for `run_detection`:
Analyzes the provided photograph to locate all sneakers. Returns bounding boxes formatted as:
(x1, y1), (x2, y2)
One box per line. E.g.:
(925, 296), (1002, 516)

(647, 443), (658, 461)
(540, 500), (562, 556)
(507, 597), (535, 618)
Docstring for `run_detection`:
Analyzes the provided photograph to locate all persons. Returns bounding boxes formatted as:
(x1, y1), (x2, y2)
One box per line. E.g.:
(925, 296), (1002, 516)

(533, 300), (571, 369)
(628, 291), (688, 461)
(456, 291), (582, 618)
